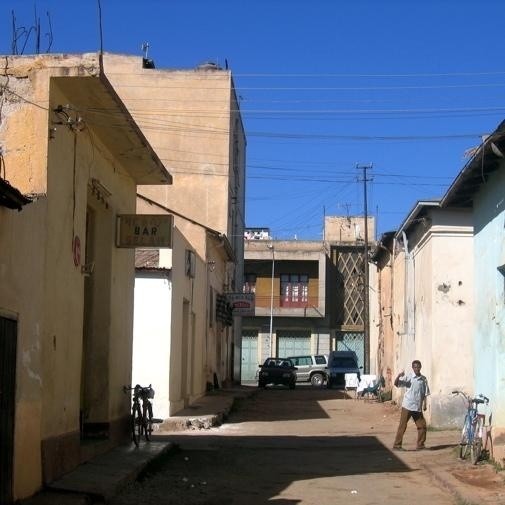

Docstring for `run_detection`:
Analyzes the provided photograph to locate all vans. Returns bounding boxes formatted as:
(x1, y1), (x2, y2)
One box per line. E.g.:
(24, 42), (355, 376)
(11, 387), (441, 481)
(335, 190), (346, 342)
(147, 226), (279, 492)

(268, 356), (328, 387)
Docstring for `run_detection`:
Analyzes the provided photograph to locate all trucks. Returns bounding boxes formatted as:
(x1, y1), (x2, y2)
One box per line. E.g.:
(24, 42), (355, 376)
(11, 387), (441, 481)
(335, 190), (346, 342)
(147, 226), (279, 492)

(325, 352), (363, 389)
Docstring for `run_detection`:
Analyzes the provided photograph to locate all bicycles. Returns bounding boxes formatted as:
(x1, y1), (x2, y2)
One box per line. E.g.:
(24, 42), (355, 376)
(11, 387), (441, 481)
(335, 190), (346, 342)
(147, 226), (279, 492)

(452, 390), (488, 466)
(123, 385), (162, 445)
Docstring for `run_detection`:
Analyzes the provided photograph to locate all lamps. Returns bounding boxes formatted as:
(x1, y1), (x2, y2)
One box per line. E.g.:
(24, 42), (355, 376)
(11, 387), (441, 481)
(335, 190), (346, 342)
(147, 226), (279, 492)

(207, 258), (216, 272)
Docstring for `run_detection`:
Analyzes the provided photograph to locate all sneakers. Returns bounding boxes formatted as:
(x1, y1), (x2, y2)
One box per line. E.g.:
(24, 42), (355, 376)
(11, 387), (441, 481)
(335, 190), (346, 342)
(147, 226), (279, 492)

(415, 446), (430, 450)
(393, 447), (406, 451)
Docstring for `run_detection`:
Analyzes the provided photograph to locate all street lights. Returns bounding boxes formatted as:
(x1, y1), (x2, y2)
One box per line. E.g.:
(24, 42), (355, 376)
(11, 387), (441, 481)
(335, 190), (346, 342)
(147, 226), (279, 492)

(261, 244), (274, 360)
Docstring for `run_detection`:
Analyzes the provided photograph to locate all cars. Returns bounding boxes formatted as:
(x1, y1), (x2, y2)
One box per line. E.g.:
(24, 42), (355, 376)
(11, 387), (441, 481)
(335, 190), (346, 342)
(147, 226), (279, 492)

(258, 357), (298, 390)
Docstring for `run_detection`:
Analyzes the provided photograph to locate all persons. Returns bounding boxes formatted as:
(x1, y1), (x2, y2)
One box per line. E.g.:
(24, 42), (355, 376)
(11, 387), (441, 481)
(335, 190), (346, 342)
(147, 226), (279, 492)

(393, 360), (431, 452)
